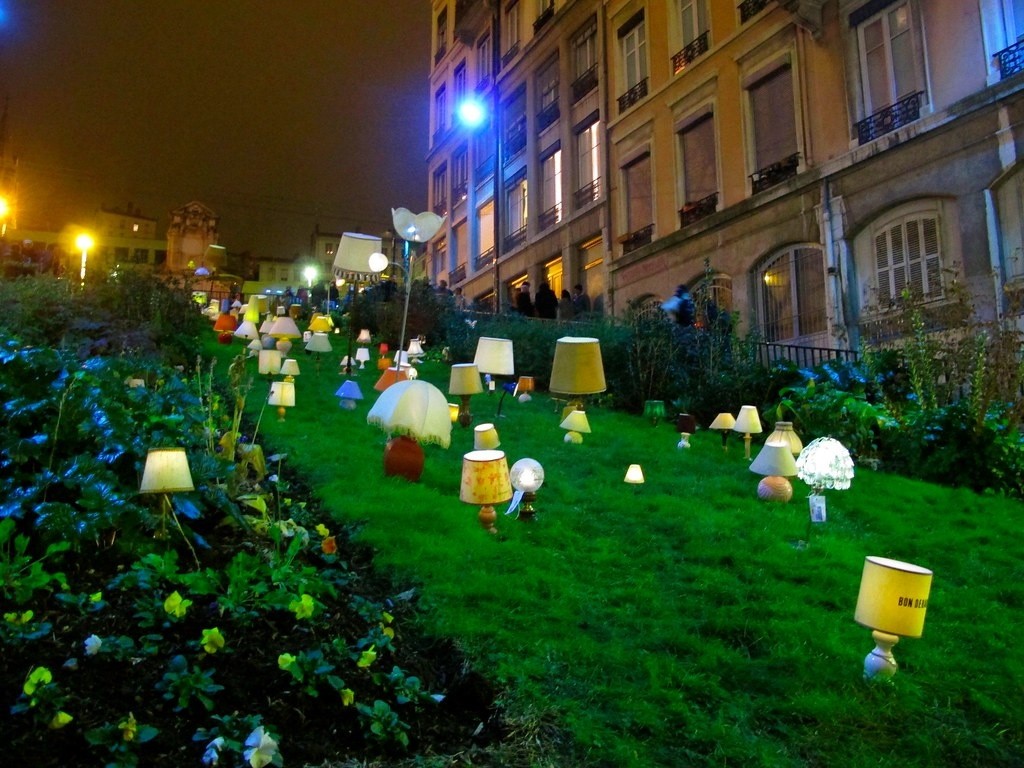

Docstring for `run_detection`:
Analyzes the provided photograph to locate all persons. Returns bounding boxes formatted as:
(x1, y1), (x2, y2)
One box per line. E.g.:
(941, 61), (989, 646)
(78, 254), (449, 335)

(660, 284), (692, 327)
(279, 279), (340, 312)
(343, 273), (591, 325)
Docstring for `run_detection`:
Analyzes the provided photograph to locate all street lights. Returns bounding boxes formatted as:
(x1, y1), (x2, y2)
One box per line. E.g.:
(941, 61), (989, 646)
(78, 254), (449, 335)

(76, 235), (92, 290)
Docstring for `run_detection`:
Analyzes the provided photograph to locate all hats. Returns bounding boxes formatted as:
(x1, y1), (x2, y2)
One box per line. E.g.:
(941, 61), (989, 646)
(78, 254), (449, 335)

(575, 284), (582, 291)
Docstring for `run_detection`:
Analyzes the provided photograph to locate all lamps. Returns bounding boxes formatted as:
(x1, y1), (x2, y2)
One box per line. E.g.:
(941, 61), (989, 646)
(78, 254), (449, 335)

(709, 413), (736, 448)
(643, 400), (665, 428)
(558, 410), (592, 444)
(624, 463), (644, 493)
(854, 556), (932, 679)
(138, 231), (545, 571)
(675, 413), (695, 450)
(734, 404), (763, 460)
(766, 421), (802, 456)
(550, 336), (607, 417)
(750, 441), (800, 503)
